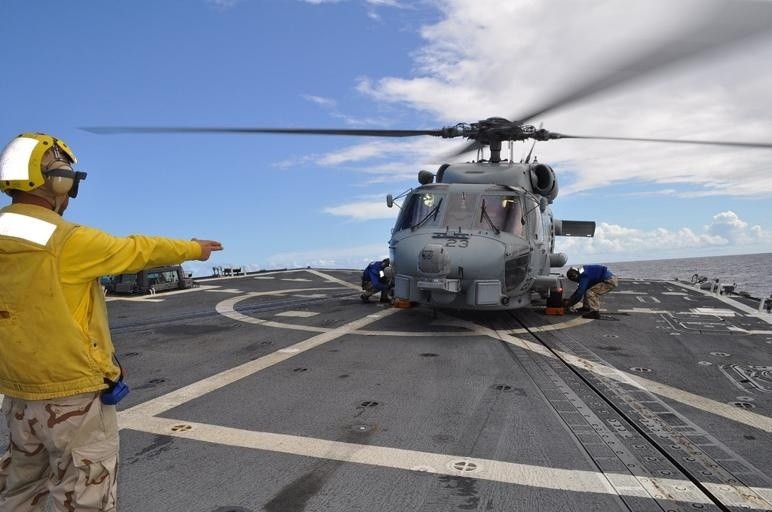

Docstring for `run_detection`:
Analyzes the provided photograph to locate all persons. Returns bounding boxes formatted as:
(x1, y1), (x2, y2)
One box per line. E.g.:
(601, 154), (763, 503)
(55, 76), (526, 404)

(0, 131), (225, 512)
(563, 265), (620, 321)
(359, 257), (396, 304)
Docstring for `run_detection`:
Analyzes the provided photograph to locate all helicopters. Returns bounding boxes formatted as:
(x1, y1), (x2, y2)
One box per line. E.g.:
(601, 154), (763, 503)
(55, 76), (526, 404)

(73, 2), (770, 312)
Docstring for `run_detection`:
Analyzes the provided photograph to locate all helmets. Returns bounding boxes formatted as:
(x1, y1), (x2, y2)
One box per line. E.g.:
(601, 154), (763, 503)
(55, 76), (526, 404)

(567, 267), (579, 282)
(0, 133), (77, 192)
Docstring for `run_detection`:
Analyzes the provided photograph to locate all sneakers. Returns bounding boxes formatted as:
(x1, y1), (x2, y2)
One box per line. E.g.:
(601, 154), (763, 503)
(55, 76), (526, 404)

(380, 298), (390, 302)
(361, 294), (368, 302)
(576, 306), (600, 319)
